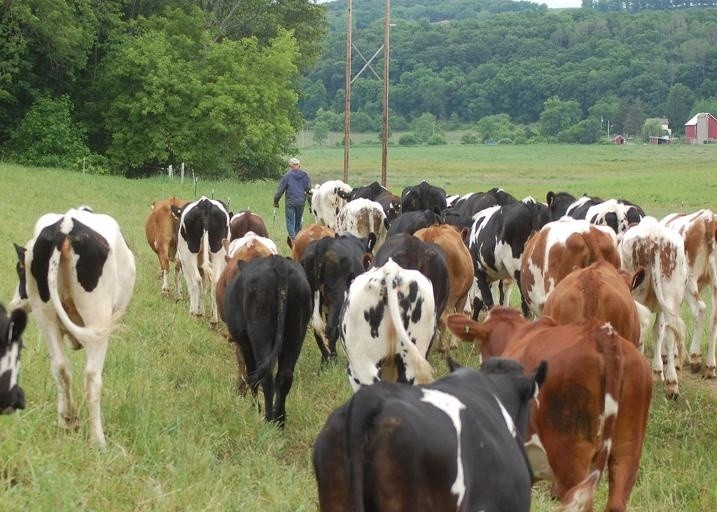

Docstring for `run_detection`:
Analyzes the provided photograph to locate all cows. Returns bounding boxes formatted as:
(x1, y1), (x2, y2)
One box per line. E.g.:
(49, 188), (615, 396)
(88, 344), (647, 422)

(412, 223), (476, 352)
(466, 195), (553, 322)
(547, 189), (646, 237)
(221, 229), (279, 260)
(300, 233), (379, 370)
(313, 355), (551, 510)
(0, 303), (30, 416)
(304, 179), (352, 228)
(338, 256), (437, 398)
(229, 207), (269, 241)
(10, 205), (138, 448)
(445, 192), (498, 218)
(543, 260), (644, 352)
(620, 215), (689, 402)
(337, 181), (403, 226)
(384, 209), (445, 242)
(335, 197), (389, 256)
(400, 180), (448, 216)
(225, 254), (316, 430)
(658, 208), (717, 383)
(487, 186), (519, 206)
(145, 194), (192, 303)
(171, 196), (232, 331)
(216, 238), (276, 399)
(446, 304), (655, 512)
(368, 232), (452, 323)
(287, 221), (336, 263)
(521, 214), (620, 323)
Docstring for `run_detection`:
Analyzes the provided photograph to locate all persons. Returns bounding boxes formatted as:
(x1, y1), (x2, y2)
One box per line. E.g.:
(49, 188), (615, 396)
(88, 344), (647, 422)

(273, 157), (312, 243)
